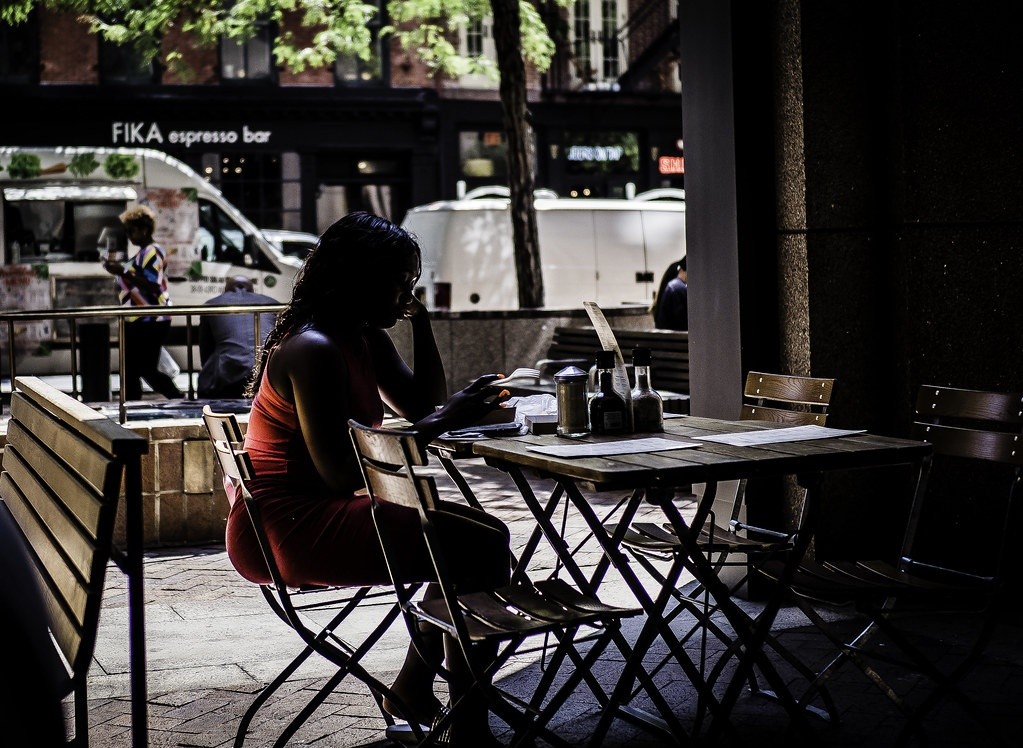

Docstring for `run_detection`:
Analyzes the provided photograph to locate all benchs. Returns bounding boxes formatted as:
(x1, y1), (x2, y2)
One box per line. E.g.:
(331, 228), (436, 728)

(0, 365), (149, 748)
(546, 324), (690, 408)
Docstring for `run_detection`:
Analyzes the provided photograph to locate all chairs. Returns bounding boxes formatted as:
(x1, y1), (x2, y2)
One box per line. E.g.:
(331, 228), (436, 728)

(197, 367), (1023, 748)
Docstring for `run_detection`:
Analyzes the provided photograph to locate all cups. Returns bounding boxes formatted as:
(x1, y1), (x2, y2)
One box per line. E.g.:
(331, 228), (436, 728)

(106, 238), (116, 262)
(432, 271), (453, 312)
(40, 243), (50, 265)
(12, 243), (21, 265)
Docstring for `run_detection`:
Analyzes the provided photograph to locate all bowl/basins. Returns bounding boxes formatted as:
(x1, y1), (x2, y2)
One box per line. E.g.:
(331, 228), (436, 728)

(435, 402), (516, 429)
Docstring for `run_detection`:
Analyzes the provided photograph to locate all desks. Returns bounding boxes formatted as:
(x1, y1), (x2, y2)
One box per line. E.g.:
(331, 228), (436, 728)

(465, 410), (927, 748)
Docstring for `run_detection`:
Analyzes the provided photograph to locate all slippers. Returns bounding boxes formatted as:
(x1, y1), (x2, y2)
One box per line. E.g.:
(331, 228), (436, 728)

(385, 706), (462, 744)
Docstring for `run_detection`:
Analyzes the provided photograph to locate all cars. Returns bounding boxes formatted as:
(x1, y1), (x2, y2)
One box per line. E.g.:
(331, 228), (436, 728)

(215, 225), (320, 274)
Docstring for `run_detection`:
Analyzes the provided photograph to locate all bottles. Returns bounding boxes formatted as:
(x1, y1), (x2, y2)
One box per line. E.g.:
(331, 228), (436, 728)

(588, 351), (630, 437)
(630, 348), (664, 435)
(554, 366), (588, 437)
(588, 349), (600, 398)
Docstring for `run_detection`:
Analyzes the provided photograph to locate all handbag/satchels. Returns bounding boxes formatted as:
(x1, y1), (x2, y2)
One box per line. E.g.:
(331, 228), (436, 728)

(157, 346), (181, 379)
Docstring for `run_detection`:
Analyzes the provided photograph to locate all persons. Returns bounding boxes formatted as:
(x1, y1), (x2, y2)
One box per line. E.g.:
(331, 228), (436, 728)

(223, 212), (512, 748)
(104, 203), (186, 402)
(652, 253), (687, 333)
(196, 275), (285, 401)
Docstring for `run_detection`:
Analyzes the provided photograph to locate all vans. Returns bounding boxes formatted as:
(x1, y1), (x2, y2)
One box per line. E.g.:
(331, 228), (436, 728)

(0, 147), (303, 377)
(398, 184), (688, 314)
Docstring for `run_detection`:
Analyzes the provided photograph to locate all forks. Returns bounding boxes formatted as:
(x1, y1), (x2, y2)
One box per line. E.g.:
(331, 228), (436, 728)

(486, 368), (540, 386)
